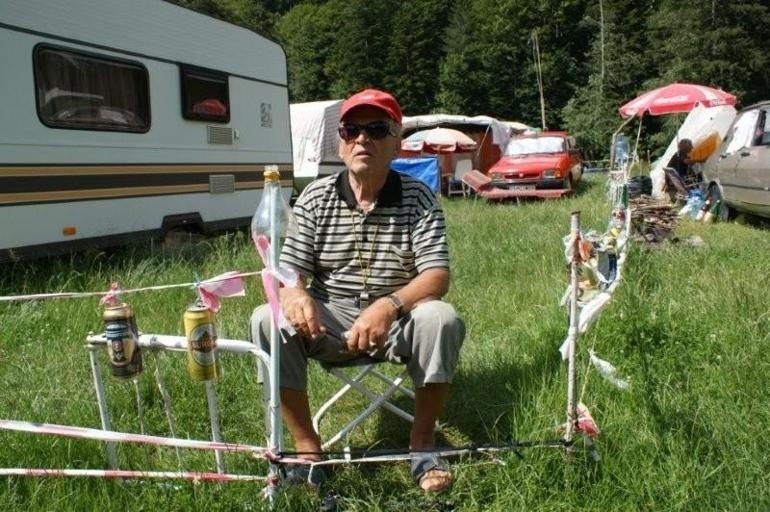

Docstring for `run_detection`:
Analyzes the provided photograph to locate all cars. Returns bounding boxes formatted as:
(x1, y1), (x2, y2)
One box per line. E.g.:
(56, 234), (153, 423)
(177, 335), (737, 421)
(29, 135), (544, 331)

(702, 100), (769, 223)
(487, 132), (583, 198)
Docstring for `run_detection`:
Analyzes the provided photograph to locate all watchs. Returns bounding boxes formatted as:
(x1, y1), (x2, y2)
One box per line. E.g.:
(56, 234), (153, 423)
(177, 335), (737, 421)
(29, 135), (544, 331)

(387, 294), (406, 321)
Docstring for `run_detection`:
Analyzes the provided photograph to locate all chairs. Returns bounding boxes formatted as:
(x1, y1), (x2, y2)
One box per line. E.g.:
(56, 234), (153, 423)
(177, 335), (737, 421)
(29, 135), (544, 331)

(663, 167), (701, 206)
(448, 159), (473, 199)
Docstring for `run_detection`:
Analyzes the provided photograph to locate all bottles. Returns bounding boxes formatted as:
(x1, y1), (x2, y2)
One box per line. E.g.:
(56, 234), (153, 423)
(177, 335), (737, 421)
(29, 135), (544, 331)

(695, 197), (720, 224)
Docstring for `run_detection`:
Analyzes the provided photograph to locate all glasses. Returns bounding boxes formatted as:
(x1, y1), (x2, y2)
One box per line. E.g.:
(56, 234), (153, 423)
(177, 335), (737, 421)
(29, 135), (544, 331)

(336, 121), (391, 140)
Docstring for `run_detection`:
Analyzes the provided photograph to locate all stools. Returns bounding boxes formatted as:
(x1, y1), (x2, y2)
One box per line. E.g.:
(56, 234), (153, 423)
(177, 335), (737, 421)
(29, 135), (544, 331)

(311, 353), (441, 456)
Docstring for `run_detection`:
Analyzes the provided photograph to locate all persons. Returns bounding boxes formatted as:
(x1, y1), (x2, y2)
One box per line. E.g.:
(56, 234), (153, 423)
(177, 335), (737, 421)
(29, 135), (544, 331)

(665, 138), (709, 203)
(248, 89), (468, 494)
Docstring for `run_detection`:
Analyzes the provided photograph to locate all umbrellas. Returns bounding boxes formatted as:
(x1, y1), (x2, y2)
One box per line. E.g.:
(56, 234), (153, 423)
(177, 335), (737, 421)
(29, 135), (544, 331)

(618, 80), (738, 174)
(399, 125), (477, 157)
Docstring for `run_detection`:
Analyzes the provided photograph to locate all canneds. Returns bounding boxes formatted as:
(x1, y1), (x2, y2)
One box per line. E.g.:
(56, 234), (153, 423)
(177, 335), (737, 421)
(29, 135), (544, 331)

(183, 304), (219, 380)
(102, 303), (142, 376)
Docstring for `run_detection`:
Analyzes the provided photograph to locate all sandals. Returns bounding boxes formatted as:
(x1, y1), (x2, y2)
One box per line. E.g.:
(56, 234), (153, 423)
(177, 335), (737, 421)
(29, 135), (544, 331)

(411, 457), (453, 491)
(284, 462), (322, 484)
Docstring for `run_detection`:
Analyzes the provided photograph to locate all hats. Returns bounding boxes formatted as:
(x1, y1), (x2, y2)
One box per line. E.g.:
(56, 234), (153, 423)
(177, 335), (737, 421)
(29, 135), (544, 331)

(338, 88), (402, 131)
